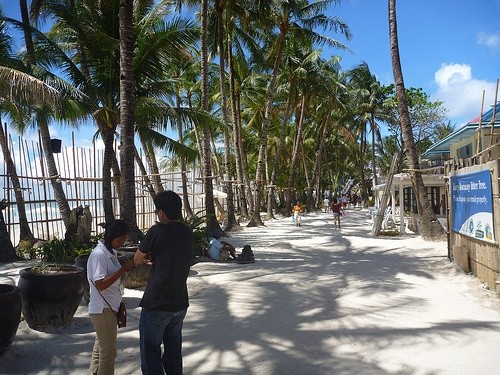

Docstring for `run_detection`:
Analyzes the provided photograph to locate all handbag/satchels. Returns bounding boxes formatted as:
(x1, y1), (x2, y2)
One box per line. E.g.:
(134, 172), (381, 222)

(117, 302), (126, 328)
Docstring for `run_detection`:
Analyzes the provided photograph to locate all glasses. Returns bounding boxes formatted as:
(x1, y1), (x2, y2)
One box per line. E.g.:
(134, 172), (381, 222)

(154, 207), (161, 215)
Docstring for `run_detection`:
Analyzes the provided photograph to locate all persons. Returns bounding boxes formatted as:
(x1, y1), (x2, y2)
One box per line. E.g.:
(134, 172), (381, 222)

(332, 199), (345, 229)
(444, 158), (454, 166)
(395, 201), (400, 206)
(133, 191), (194, 375)
(291, 201), (303, 227)
(208, 230), (235, 262)
(87, 220), (135, 375)
(323, 190), (362, 213)
(437, 194), (446, 218)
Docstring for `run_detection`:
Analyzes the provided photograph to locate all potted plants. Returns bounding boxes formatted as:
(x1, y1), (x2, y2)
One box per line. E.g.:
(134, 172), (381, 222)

(0, 233), (152, 358)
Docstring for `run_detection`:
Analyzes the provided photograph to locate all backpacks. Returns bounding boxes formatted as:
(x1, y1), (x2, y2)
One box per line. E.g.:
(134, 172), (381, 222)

(237, 245), (255, 264)
